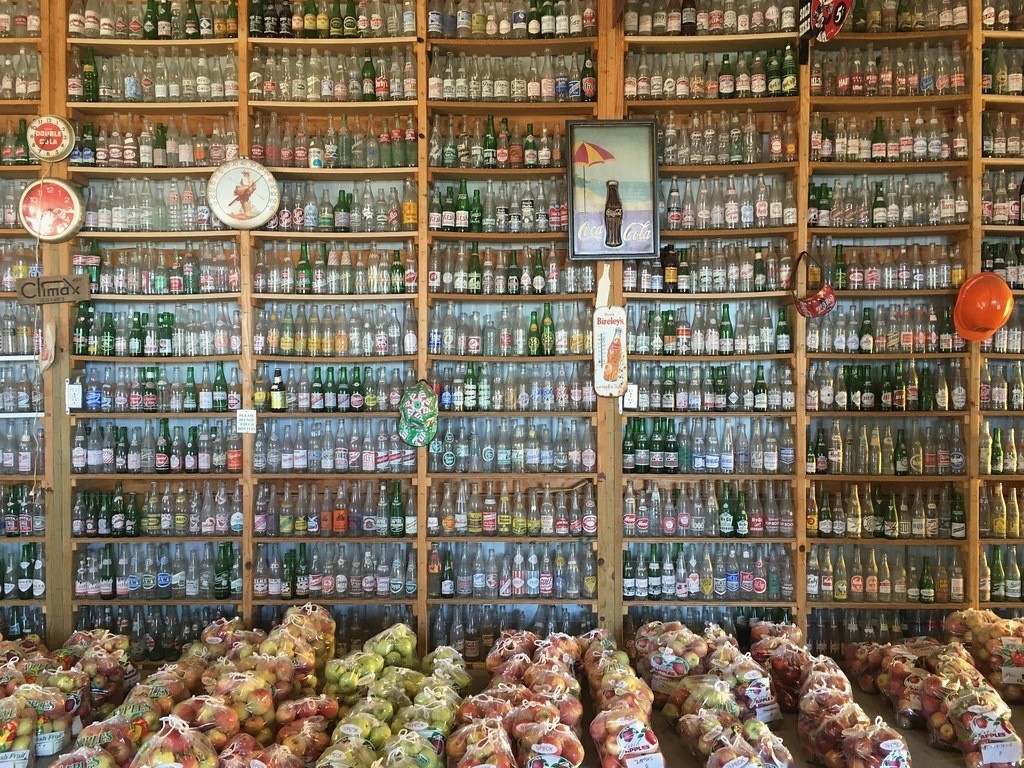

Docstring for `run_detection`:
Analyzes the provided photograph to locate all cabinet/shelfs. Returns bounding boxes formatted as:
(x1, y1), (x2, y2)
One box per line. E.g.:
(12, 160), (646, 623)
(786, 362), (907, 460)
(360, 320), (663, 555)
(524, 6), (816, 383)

(0, 0), (1024, 666)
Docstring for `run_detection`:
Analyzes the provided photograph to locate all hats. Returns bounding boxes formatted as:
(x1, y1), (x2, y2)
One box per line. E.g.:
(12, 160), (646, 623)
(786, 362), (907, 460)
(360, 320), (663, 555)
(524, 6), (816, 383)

(396, 380), (438, 446)
(790, 251), (835, 318)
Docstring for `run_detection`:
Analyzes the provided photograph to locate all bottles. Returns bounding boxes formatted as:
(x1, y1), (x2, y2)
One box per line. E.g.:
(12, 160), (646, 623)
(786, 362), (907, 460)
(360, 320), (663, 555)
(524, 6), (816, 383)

(0, 0), (1023, 670)
(603, 178), (624, 246)
(604, 327), (622, 383)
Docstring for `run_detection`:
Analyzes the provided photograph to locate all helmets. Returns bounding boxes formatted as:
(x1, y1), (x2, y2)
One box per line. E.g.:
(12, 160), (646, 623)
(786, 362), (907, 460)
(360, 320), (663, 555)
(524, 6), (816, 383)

(954, 272), (1014, 341)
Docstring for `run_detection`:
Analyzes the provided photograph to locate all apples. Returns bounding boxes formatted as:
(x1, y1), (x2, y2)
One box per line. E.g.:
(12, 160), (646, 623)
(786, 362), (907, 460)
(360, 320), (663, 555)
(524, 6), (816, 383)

(0, 607), (1024, 768)
